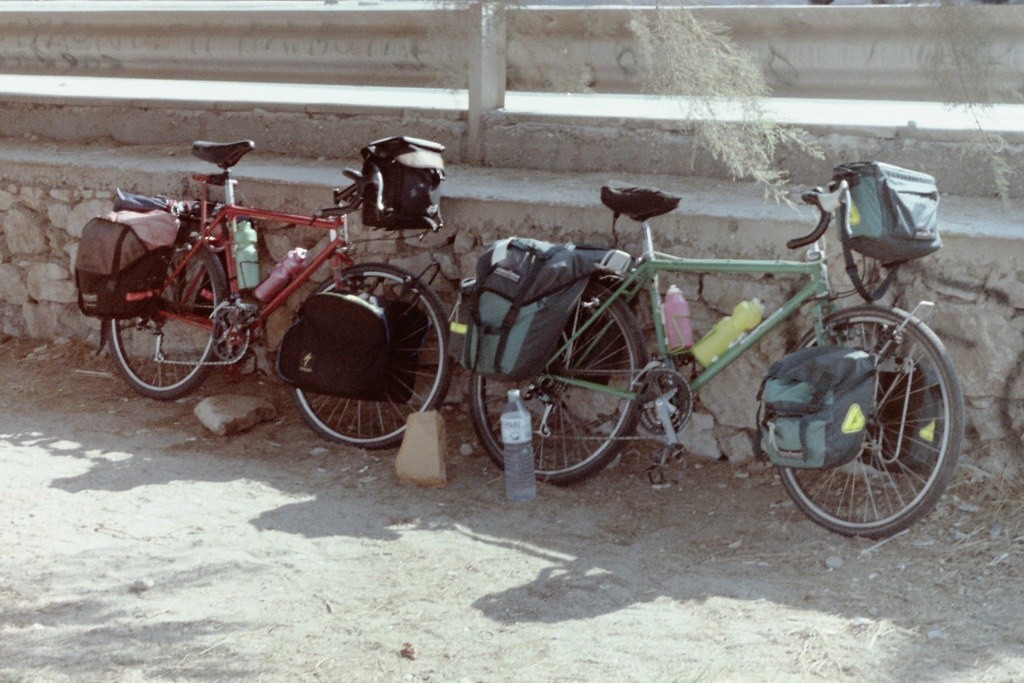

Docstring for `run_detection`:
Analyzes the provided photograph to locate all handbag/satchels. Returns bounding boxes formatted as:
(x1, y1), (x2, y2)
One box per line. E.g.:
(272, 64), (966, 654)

(362, 137), (446, 229)
(74, 188), (256, 319)
(828, 162), (943, 302)
(446, 239), (639, 384)
(276, 285), (429, 404)
(759, 346), (950, 471)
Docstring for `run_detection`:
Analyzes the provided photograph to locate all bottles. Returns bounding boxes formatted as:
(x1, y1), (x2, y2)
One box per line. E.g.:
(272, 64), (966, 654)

(253, 246), (308, 303)
(234, 220), (261, 290)
(664, 282), (694, 353)
(500, 389), (536, 501)
(688, 296), (768, 370)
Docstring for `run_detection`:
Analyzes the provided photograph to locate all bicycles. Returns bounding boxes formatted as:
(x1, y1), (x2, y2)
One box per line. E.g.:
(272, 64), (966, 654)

(458, 158), (965, 538)
(75, 133), (453, 453)
